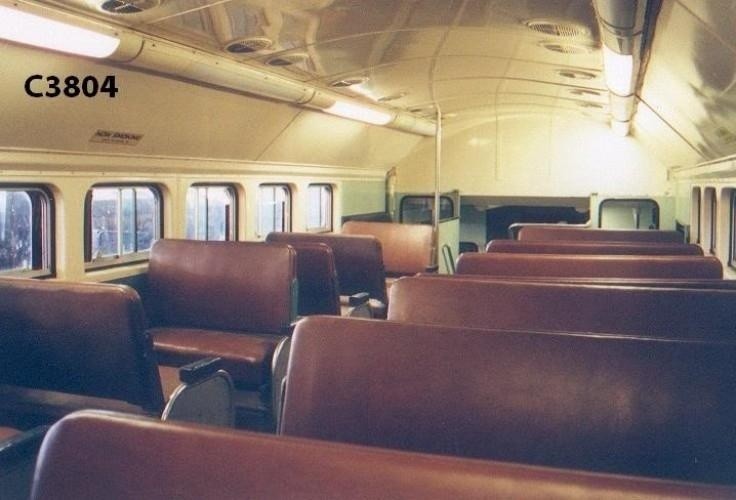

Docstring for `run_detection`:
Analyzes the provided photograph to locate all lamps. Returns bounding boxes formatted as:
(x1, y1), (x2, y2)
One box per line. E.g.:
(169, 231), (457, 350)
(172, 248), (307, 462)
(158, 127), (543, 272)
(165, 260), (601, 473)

(591, 1), (660, 143)
(0, 0), (439, 136)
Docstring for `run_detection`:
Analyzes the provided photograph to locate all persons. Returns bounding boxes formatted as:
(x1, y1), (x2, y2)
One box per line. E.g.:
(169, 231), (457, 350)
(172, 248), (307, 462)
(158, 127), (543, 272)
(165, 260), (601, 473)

(558, 207), (589, 226)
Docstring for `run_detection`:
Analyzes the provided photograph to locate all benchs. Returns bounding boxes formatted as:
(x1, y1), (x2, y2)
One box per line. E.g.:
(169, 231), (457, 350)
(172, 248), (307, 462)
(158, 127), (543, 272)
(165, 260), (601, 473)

(0, 219), (736, 500)
